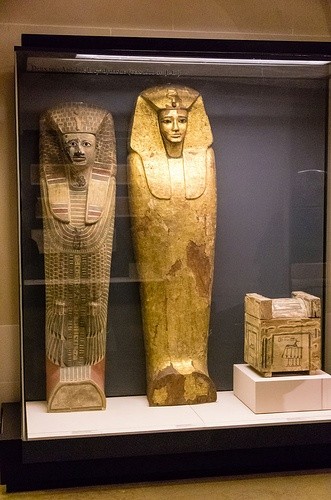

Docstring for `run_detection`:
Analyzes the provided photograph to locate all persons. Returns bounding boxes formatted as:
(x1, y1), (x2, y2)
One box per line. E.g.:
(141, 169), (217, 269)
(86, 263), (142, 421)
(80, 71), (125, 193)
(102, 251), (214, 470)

(125, 84), (218, 409)
(36, 104), (118, 413)
